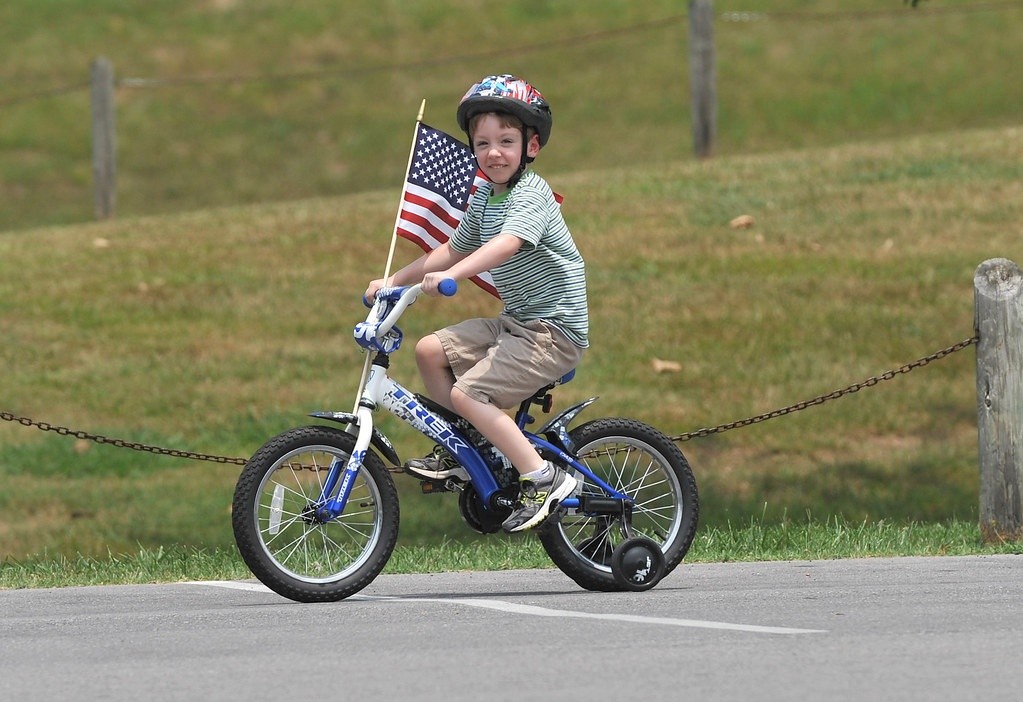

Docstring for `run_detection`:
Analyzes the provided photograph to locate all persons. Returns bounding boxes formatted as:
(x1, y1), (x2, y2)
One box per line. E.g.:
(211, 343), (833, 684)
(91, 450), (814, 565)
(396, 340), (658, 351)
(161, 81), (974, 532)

(365, 74), (589, 532)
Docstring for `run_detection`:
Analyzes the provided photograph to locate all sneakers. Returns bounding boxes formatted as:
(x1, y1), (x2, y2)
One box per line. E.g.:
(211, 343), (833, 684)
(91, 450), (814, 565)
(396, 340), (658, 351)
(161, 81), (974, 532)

(502, 461), (578, 534)
(404, 444), (472, 483)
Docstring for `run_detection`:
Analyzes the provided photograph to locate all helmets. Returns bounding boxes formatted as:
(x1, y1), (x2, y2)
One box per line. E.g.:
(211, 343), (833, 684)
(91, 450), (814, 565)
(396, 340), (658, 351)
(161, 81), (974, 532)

(456, 74), (552, 148)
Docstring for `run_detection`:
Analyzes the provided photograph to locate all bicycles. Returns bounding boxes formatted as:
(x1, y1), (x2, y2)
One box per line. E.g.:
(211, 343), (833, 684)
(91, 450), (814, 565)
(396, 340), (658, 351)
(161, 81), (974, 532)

(230, 278), (701, 603)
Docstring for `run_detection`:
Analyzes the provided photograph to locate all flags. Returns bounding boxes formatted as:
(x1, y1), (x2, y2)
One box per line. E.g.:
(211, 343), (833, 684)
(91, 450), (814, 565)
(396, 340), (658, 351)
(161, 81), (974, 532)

(397, 121), (563, 300)
